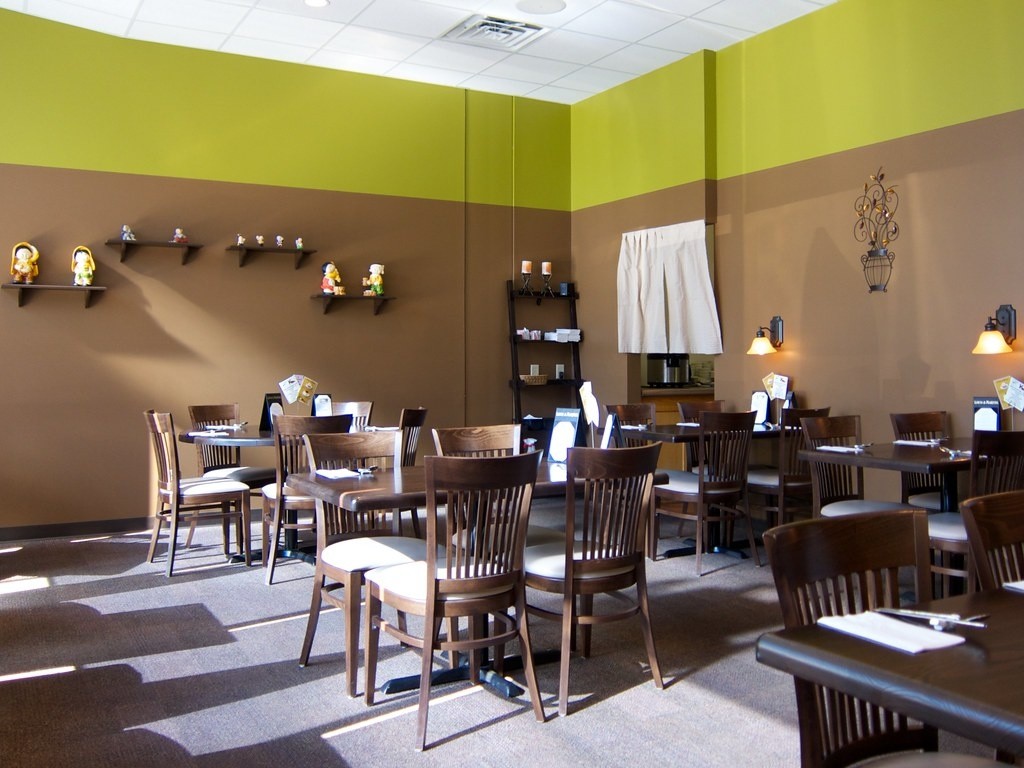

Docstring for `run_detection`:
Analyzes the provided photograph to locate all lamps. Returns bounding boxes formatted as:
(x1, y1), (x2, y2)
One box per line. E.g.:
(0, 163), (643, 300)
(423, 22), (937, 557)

(972, 304), (1017, 357)
(747, 315), (784, 356)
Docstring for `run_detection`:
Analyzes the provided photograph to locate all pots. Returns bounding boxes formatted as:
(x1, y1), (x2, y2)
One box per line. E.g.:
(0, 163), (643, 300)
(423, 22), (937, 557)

(646, 352), (693, 384)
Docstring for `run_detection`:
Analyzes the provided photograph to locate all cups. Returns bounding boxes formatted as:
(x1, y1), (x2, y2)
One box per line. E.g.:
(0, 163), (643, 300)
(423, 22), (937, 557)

(542, 261), (551, 275)
(522, 261), (531, 273)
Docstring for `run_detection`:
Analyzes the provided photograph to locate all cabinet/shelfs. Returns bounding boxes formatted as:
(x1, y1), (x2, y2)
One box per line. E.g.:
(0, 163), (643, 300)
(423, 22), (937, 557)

(507, 280), (589, 448)
(3, 239), (400, 317)
(641, 386), (715, 517)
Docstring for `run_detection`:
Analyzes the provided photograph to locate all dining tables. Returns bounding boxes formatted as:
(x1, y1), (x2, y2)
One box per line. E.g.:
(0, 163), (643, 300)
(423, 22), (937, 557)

(179, 426), (400, 566)
(598, 421), (805, 557)
(286, 455), (669, 698)
(755, 579), (1024, 757)
(797, 438), (1011, 598)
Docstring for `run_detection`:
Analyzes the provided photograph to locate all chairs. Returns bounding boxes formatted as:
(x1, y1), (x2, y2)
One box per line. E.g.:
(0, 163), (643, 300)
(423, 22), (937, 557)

(361, 406), (427, 541)
(332, 400), (375, 433)
(604, 403), (693, 557)
(261, 414), (354, 585)
(724, 406), (832, 552)
(929, 430), (1024, 600)
(675, 399), (724, 540)
(364, 447), (548, 752)
(296, 428), (459, 700)
(493, 439), (665, 718)
(184, 403), (288, 554)
(762, 508), (1017, 768)
(429, 423), (567, 643)
(800, 416), (928, 520)
(144, 408), (252, 578)
(890, 412), (969, 514)
(960, 490), (1024, 592)
(650, 409), (762, 577)
